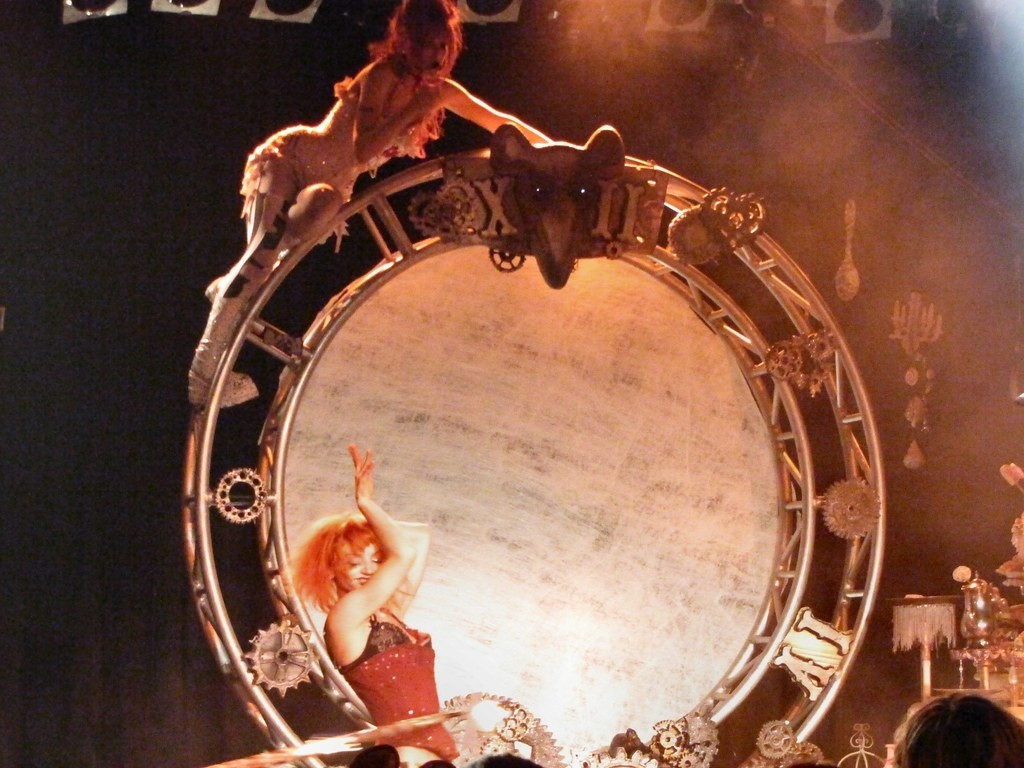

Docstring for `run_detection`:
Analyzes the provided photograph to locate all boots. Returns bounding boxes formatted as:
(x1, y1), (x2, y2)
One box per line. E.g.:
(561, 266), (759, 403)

(188, 297), (260, 407)
(205, 229), (303, 304)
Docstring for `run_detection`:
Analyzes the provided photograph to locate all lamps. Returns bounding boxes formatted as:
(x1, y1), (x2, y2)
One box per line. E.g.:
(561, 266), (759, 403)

(150, 1), (221, 17)
(456, 0), (521, 22)
(244, 1), (324, 25)
(644, 0), (715, 33)
(63, 0), (129, 22)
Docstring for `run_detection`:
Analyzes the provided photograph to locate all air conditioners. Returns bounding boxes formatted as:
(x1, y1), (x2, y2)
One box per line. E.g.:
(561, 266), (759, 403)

(825, 0), (891, 45)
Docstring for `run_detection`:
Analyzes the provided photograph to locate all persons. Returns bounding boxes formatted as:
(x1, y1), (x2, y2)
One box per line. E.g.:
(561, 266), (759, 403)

(188, 0), (553, 410)
(295, 445), (551, 768)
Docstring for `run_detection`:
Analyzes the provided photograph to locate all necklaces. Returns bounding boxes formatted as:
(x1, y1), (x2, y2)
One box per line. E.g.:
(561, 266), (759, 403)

(788, 687), (1024, 768)
